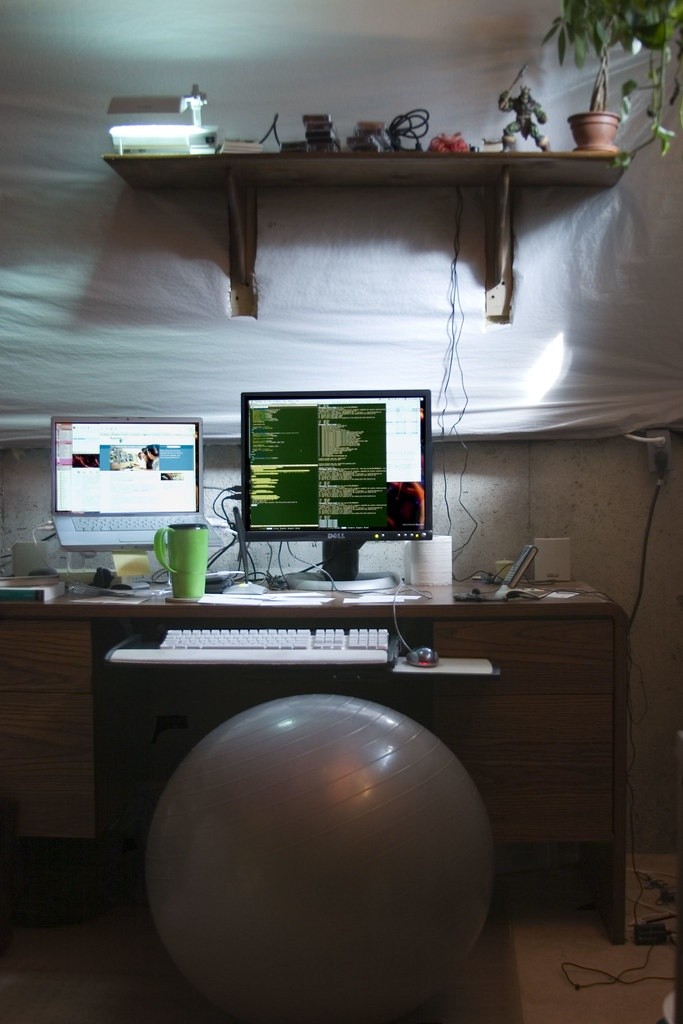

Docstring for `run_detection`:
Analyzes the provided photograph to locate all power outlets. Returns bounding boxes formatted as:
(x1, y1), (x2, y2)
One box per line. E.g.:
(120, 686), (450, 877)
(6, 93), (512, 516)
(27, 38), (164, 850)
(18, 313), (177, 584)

(646, 430), (672, 475)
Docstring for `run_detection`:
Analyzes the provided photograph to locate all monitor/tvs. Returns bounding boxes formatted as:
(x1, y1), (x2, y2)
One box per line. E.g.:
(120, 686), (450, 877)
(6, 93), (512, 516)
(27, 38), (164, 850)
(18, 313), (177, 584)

(239, 390), (434, 593)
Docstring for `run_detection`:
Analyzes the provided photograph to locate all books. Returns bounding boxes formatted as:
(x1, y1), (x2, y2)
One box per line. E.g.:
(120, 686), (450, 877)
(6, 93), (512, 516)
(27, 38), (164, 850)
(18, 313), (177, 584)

(0, 576), (66, 602)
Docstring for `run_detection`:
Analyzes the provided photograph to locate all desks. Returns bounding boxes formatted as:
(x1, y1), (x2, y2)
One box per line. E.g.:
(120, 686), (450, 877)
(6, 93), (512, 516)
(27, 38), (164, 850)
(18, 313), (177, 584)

(0, 579), (631, 943)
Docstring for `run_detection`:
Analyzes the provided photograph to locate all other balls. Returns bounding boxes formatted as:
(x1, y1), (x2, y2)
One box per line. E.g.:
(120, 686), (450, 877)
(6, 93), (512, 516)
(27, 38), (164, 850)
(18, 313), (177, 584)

(143, 693), (496, 1024)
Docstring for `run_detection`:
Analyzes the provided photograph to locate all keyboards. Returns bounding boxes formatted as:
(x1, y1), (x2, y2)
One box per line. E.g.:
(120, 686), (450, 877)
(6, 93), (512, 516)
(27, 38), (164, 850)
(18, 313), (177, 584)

(159, 628), (389, 663)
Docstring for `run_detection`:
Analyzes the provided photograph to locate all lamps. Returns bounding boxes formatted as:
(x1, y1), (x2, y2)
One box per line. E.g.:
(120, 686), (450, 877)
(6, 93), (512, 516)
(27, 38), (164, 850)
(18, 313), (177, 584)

(107, 85), (218, 155)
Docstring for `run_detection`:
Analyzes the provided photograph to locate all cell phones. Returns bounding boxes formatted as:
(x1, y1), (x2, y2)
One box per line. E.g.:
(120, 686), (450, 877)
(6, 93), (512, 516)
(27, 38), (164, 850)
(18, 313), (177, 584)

(502, 545), (539, 587)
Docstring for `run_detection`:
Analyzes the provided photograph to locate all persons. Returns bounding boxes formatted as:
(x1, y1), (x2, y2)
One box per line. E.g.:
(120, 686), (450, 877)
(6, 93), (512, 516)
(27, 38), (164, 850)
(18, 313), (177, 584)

(131, 445), (160, 471)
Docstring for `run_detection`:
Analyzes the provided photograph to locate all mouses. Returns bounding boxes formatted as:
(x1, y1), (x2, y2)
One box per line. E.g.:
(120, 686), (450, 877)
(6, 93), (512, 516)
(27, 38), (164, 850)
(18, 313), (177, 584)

(407, 646), (440, 667)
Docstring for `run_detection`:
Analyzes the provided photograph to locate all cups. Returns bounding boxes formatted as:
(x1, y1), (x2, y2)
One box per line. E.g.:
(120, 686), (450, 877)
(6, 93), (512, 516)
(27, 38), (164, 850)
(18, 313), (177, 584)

(154, 523), (209, 598)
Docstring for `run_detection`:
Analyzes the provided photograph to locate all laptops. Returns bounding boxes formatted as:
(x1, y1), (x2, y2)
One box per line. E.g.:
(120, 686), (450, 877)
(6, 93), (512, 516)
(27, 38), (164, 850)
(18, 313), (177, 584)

(49, 416), (222, 551)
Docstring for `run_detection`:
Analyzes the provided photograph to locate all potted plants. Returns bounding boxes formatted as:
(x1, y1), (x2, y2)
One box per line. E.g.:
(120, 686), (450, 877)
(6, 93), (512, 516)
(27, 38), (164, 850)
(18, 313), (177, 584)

(539, 0), (683, 168)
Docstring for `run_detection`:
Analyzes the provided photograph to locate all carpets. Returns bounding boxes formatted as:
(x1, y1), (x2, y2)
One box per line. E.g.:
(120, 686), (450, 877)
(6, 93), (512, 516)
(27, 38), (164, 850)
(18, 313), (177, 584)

(0, 875), (524, 1024)
